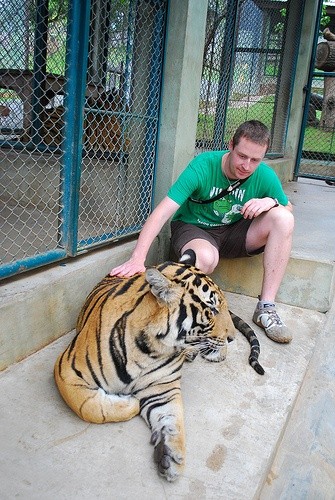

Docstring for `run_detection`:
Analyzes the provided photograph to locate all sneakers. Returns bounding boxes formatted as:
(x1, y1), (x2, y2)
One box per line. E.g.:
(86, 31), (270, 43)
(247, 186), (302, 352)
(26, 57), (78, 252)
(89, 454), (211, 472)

(252, 300), (292, 343)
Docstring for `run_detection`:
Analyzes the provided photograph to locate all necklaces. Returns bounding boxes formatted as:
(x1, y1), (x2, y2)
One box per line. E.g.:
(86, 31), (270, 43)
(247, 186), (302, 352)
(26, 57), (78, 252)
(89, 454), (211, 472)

(224, 152), (241, 192)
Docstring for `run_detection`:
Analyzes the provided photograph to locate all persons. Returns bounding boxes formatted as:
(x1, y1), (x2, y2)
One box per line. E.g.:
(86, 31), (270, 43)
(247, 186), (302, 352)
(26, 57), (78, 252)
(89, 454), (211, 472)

(109, 119), (294, 345)
